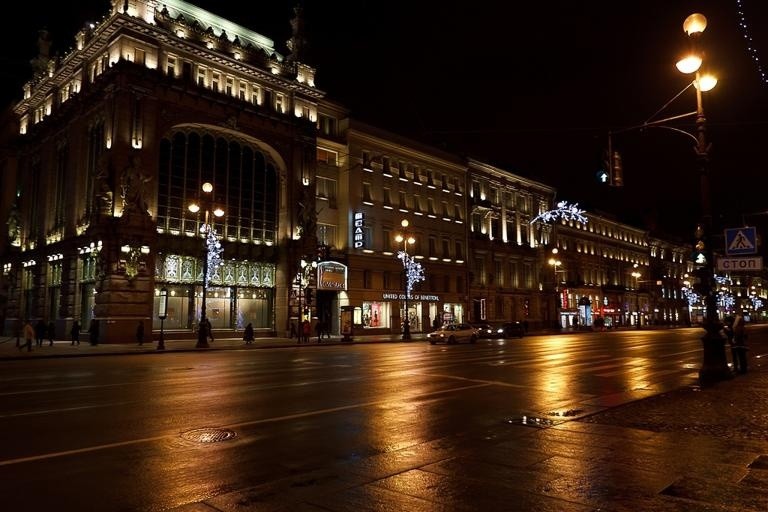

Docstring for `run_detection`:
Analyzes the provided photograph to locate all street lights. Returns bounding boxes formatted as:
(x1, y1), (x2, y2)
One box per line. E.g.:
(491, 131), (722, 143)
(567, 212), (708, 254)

(394, 220), (416, 340)
(548, 248), (561, 329)
(188, 183), (224, 347)
(675, 11), (721, 388)
(632, 264), (641, 327)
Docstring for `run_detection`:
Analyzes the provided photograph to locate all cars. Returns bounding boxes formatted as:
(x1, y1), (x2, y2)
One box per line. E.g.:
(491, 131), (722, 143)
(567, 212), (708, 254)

(479, 321), (524, 337)
(427, 323), (480, 344)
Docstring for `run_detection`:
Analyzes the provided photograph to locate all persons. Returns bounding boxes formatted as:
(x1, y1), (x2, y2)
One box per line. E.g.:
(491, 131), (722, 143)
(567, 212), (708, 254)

(71, 321), (82, 346)
(289, 319), (331, 343)
(593, 317), (605, 332)
(19, 319), (56, 352)
(205, 318), (214, 342)
(433, 317), (438, 331)
(243, 323), (256, 344)
(723, 316), (752, 374)
(136, 320), (144, 346)
(573, 317), (579, 330)
(519, 319), (529, 333)
(87, 318), (99, 346)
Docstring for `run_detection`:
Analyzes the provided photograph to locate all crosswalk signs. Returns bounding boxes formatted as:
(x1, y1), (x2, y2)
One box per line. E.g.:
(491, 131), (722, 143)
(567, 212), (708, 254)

(725, 228), (756, 253)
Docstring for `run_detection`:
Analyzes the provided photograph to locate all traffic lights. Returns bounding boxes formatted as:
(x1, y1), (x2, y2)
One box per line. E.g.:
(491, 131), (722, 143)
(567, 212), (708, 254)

(597, 149), (610, 182)
